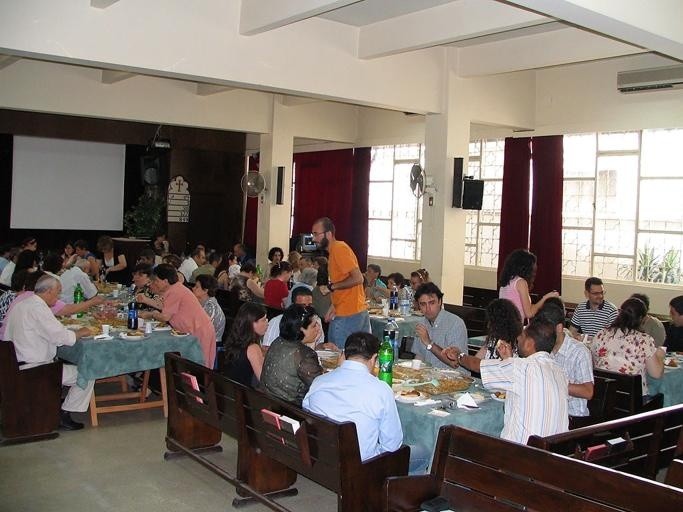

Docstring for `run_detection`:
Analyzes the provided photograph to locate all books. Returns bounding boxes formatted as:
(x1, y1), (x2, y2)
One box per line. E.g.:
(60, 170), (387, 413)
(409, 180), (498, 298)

(279, 415), (302, 451)
(178, 373), (203, 403)
(606, 437), (630, 456)
(259, 408), (284, 447)
(583, 444), (608, 460)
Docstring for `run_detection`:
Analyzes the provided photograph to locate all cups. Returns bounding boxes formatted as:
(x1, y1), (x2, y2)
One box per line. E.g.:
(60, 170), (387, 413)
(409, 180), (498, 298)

(113, 290), (118, 297)
(661, 347), (667, 354)
(102, 324), (110, 335)
(71, 314), (77, 320)
(144, 322), (152, 333)
(117, 284), (122, 291)
(412, 359), (425, 370)
(381, 299), (387, 305)
(138, 318), (144, 327)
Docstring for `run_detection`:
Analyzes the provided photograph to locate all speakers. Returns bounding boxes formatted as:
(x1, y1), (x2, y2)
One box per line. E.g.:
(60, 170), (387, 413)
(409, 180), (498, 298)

(462, 175), (484, 210)
(452, 157), (464, 208)
(275, 166), (285, 206)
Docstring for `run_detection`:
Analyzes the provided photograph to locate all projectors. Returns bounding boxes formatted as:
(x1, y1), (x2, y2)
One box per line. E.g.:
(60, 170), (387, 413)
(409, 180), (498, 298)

(146, 138), (172, 154)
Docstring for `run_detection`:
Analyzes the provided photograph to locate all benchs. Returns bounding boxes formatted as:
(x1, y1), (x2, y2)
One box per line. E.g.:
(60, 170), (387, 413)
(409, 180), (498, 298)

(443, 304), (486, 338)
(397, 336), (616, 430)
(464, 286), (539, 310)
(162, 352), (410, 512)
(215, 288), (329, 344)
(383, 424), (683, 512)
(467, 343), (664, 419)
(0, 339), (63, 446)
(527, 403), (683, 480)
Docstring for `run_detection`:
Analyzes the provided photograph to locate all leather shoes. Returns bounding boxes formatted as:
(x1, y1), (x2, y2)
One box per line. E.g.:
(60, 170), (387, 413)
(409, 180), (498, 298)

(59, 412), (84, 430)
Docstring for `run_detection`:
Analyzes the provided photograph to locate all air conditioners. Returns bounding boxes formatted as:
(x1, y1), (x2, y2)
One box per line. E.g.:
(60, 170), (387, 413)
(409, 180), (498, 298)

(617, 62), (683, 95)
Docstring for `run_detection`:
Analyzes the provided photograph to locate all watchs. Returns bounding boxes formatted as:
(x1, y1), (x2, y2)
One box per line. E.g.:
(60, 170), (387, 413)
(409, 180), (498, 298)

(457, 352), (466, 366)
(327, 283), (334, 292)
(425, 340), (435, 350)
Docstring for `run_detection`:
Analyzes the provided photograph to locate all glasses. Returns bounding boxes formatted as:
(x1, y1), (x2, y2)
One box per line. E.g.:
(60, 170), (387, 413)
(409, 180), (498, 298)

(311, 231), (328, 236)
(588, 290), (607, 296)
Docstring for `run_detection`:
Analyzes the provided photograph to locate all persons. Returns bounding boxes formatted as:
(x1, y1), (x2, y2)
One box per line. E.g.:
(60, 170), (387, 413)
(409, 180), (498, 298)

(376, 272), (411, 303)
(457, 298), (523, 368)
(529, 301), (598, 431)
(95, 237), (130, 285)
(221, 303), (270, 387)
(4, 275), (95, 432)
(162, 255), (187, 286)
(663, 294), (683, 353)
(138, 249), (157, 269)
(305, 333), (403, 460)
(498, 249), (559, 322)
(364, 264), (387, 299)
(312, 265), (332, 318)
(408, 269), (431, 312)
(263, 261), (293, 308)
(410, 282), (472, 376)
(146, 231), (170, 255)
(313, 256), (327, 269)
(294, 257), (313, 283)
(289, 250), (302, 282)
(0, 272), (29, 323)
(259, 304), (325, 407)
(0, 270), (104, 341)
(183, 243), (263, 297)
(568, 276), (618, 341)
(0, 234), (94, 273)
(260, 286), (341, 351)
(444, 318), (569, 447)
(261, 247), (284, 280)
(136, 263), (217, 371)
(627, 291), (666, 348)
(285, 268), (318, 310)
(60, 260), (99, 317)
(311, 217), (371, 350)
(592, 298), (667, 412)
(132, 264), (157, 301)
(190, 274), (226, 343)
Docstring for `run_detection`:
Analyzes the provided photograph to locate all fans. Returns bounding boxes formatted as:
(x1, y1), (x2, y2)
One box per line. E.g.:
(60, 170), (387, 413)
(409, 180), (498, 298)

(242, 170), (267, 206)
(410, 162), (436, 208)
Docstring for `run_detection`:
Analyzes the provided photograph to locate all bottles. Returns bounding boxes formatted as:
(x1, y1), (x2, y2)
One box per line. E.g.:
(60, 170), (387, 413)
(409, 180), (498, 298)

(254, 264), (262, 274)
(378, 336), (394, 388)
(98, 264), (107, 288)
(127, 296), (139, 329)
(400, 284), (410, 315)
(389, 282), (398, 309)
(383, 317), (394, 360)
(391, 318), (399, 364)
(74, 283), (84, 318)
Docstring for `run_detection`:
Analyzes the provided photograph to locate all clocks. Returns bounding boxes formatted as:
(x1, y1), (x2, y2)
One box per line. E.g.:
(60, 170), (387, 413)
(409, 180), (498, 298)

(141, 156), (161, 199)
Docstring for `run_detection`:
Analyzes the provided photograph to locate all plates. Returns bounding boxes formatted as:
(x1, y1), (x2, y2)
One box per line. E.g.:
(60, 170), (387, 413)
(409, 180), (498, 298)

(123, 333), (144, 340)
(153, 326), (172, 331)
(315, 350), (334, 358)
(61, 321), (85, 327)
(454, 393), (485, 404)
(491, 393), (506, 403)
(171, 333), (190, 337)
(391, 379), (405, 386)
(395, 391), (424, 404)
(144, 321), (161, 324)
(436, 368), (466, 379)
(406, 379), (428, 386)
(81, 337), (93, 340)
(664, 365), (681, 369)
